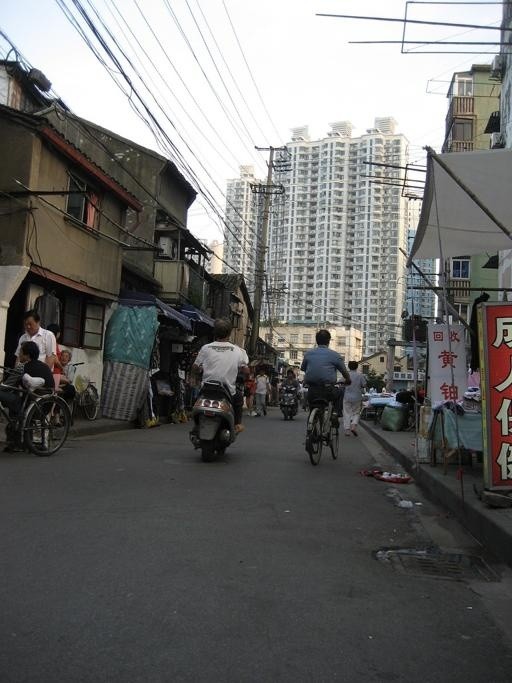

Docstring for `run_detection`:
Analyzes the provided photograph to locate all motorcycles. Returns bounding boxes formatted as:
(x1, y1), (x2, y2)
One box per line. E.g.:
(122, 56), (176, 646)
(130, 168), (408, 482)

(188, 376), (253, 462)
(279, 384), (299, 421)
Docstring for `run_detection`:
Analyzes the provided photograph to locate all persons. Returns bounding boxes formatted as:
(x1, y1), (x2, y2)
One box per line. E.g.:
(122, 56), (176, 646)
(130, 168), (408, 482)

(192, 316), (250, 432)
(244, 360), (407, 435)
(300, 328), (352, 452)
(4, 308), (77, 453)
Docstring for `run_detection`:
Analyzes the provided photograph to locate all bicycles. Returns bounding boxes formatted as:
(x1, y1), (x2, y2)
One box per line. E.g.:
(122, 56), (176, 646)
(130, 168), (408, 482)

(303, 391), (308, 412)
(373, 405), (384, 426)
(0, 360), (101, 456)
(300, 380), (347, 468)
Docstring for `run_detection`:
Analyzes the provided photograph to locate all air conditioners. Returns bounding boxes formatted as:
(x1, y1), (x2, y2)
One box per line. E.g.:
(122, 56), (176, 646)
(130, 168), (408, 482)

(490, 132), (504, 147)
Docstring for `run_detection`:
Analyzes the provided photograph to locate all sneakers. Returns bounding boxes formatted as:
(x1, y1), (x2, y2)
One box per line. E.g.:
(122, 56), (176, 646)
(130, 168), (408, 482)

(235, 423), (245, 433)
(330, 412), (340, 429)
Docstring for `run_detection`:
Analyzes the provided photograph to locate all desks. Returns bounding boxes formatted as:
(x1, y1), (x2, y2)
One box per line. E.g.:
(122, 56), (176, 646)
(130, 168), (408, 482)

(426, 407), (483, 477)
